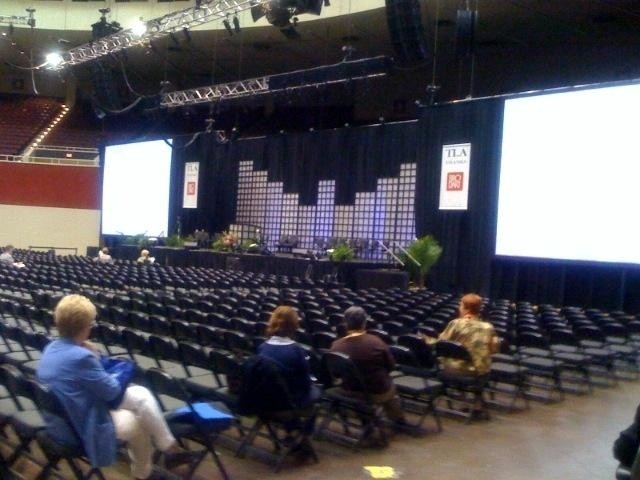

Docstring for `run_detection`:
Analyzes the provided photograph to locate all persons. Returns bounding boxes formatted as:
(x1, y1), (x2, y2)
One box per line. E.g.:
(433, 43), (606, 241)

(330, 306), (407, 442)
(137, 250), (155, 266)
(254, 305), (321, 457)
(99, 247), (113, 265)
(0, 245), (21, 269)
(193, 229), (201, 240)
(419, 294), (503, 414)
(35, 294), (204, 480)
(200, 228), (209, 249)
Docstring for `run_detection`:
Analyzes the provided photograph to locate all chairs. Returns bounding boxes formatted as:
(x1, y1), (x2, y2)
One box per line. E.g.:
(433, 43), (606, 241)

(2, 266), (348, 475)
(340, 286), (640, 452)
(1, 247), (163, 266)
(0, 91), (104, 166)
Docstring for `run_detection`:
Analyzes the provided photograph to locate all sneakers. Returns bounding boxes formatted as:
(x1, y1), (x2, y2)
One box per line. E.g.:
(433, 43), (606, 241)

(136, 470), (182, 480)
(165, 447), (202, 470)
